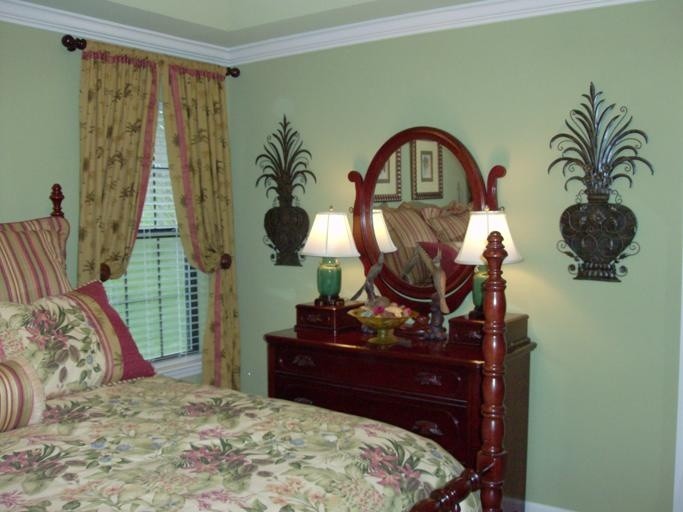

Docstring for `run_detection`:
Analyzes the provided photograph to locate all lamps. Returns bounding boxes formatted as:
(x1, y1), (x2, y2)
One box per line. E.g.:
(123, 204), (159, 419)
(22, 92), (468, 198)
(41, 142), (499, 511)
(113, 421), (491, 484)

(301, 205), (362, 306)
(371, 209), (398, 254)
(454, 205), (523, 311)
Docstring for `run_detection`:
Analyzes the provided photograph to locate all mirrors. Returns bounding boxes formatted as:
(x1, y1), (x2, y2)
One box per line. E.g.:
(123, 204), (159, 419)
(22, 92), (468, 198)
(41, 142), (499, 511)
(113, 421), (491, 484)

(348, 126), (506, 314)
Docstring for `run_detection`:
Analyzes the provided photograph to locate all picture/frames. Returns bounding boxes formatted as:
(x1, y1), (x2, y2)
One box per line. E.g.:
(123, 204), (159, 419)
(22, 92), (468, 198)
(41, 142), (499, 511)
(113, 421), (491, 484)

(373, 146), (401, 202)
(410, 139), (444, 200)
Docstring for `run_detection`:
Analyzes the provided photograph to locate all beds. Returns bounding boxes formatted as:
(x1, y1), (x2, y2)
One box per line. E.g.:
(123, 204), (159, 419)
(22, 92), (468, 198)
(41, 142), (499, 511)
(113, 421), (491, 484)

(0, 184), (508, 512)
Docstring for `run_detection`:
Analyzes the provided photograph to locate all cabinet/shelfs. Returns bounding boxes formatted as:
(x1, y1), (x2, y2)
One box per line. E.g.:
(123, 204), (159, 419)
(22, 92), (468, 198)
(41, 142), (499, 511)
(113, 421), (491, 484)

(264, 328), (537, 512)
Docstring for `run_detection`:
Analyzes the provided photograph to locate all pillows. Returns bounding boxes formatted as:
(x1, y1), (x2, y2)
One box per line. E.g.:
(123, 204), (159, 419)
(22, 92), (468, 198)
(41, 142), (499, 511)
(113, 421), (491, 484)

(0, 280), (155, 400)
(0, 217), (74, 304)
(0, 357), (46, 433)
(380, 201), (473, 286)
